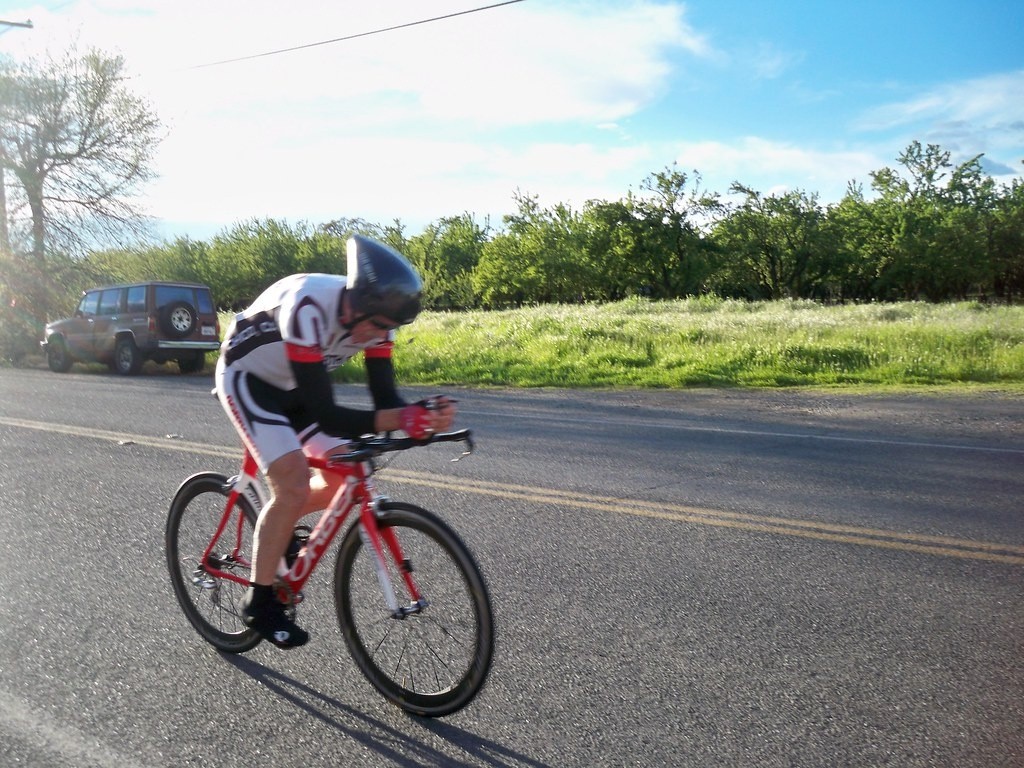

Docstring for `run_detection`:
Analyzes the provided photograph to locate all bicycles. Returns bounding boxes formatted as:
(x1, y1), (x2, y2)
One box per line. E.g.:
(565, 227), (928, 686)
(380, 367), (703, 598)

(165, 429), (496, 717)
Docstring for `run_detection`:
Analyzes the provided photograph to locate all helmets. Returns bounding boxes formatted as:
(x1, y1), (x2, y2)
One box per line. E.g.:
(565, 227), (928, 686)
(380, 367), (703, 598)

(346, 234), (423, 324)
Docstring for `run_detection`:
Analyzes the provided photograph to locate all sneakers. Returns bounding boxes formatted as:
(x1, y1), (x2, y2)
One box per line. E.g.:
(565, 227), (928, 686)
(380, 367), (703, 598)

(242, 600), (309, 648)
(284, 525), (313, 570)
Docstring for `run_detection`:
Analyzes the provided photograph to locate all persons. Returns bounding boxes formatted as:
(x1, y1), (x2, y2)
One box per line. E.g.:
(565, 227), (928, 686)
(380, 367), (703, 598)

(215, 236), (453, 649)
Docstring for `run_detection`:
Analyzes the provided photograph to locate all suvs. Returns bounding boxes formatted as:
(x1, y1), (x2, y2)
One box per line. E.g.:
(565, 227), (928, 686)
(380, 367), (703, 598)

(39, 280), (222, 375)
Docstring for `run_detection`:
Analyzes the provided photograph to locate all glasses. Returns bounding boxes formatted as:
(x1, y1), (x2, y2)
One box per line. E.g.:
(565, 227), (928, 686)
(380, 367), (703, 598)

(369, 320), (397, 332)
